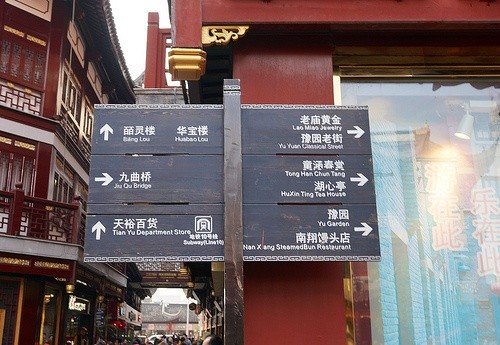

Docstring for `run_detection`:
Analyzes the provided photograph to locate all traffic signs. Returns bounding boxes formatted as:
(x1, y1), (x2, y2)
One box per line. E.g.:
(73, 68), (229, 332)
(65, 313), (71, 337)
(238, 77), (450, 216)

(83, 104), (382, 262)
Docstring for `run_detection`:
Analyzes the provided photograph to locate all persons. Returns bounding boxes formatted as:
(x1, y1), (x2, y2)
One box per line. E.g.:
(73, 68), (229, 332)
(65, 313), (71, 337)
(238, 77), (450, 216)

(41, 324), (224, 345)
